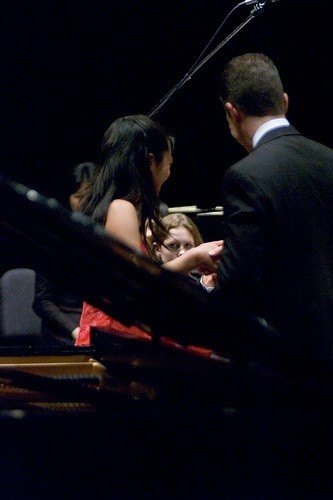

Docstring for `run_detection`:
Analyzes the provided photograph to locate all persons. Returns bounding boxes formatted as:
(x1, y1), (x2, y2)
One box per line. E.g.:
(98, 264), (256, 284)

(146, 213), (203, 265)
(32, 161), (104, 345)
(212, 53), (333, 370)
(75, 114), (225, 279)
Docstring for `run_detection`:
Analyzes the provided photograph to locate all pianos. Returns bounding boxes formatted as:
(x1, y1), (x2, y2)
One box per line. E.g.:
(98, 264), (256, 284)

(0, 179), (236, 415)
